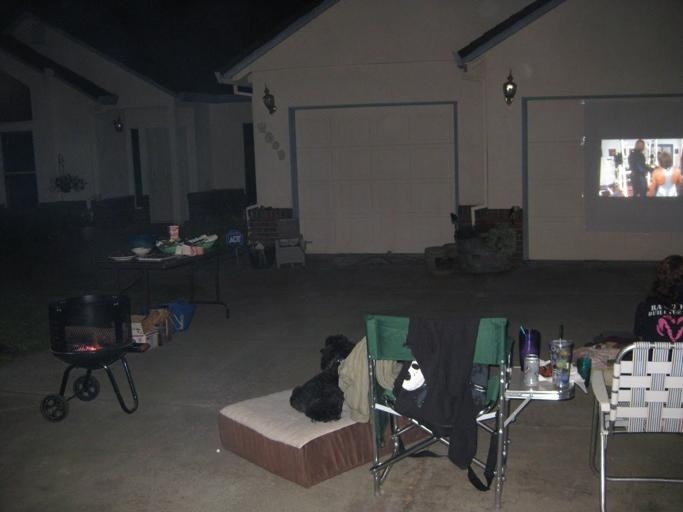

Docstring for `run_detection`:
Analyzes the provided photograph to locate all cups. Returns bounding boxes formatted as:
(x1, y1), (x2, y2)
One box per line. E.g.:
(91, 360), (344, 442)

(519, 329), (540, 369)
(577, 357), (592, 387)
(549, 338), (573, 391)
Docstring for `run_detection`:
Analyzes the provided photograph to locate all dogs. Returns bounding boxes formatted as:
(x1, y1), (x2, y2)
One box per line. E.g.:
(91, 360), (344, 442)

(288, 332), (359, 424)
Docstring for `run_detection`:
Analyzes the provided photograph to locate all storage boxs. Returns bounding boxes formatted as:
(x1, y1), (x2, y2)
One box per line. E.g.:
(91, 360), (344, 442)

(130, 308), (169, 351)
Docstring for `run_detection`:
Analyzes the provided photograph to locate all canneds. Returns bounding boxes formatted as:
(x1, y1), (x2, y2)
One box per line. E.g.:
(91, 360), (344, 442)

(523, 354), (540, 387)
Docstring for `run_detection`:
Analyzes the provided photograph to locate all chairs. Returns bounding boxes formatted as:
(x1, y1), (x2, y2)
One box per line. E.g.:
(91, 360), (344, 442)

(363, 313), (515, 510)
(587, 340), (681, 510)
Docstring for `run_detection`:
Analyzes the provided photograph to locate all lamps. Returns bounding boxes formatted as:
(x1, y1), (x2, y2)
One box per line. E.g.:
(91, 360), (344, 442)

(501, 69), (517, 106)
(113, 113), (125, 135)
(261, 81), (278, 114)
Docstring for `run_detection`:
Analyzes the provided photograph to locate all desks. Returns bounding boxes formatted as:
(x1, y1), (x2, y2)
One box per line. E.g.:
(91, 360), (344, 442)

(502, 369), (576, 429)
(94, 233), (234, 320)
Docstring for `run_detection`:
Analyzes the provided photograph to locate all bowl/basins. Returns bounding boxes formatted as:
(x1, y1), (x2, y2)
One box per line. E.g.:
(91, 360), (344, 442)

(131, 247), (152, 256)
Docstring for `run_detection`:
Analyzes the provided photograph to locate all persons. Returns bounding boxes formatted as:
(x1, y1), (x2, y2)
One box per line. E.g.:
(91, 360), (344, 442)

(629, 140), (650, 197)
(631, 253), (682, 362)
(647, 153), (682, 197)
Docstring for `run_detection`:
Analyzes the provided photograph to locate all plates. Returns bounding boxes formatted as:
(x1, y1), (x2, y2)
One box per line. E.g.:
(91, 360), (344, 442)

(109, 256), (134, 262)
